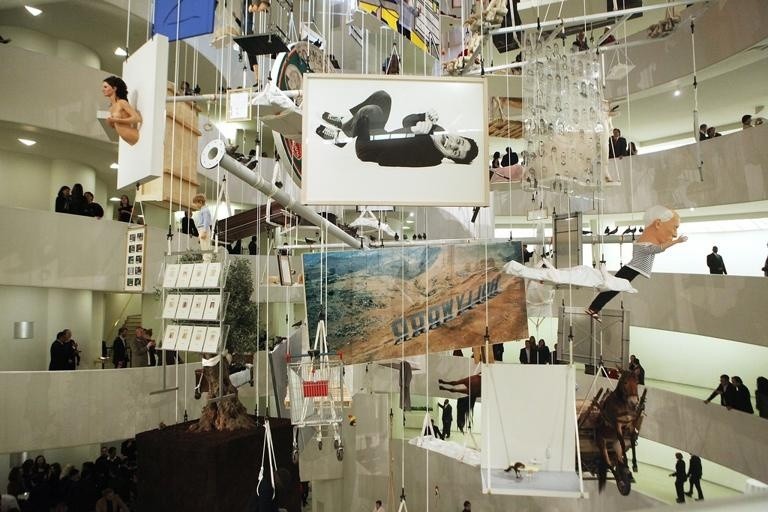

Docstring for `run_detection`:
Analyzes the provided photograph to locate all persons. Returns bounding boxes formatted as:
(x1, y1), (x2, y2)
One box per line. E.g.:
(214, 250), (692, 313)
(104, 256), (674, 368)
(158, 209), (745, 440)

(292, 275), (304, 287)
(699, 114), (765, 142)
(489, 128), (640, 169)
(394, 231), (427, 241)
(52, 182), (133, 222)
(755, 376), (767, 419)
(704, 374), (754, 414)
(761, 248), (767, 275)
(463, 501), (471, 512)
(493, 343), (504, 361)
(249, 233), (257, 255)
(519, 336), (569, 365)
(191, 194), (211, 263)
(707, 246), (727, 274)
(47, 324), (183, 371)
(584, 206), (688, 324)
(522, 243), (533, 262)
(509, 24), (627, 61)
(424, 419), (444, 440)
(0, 446), (136, 512)
(244, 0), (270, 82)
(629, 355), (644, 385)
(669, 452), (704, 503)
(371, 500), (385, 510)
(315, 90), (478, 167)
(102, 77), (143, 148)
(438, 399), (452, 438)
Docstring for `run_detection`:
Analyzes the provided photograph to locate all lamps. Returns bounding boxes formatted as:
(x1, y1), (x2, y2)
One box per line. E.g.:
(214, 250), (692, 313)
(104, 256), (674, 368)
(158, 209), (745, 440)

(14, 321), (33, 339)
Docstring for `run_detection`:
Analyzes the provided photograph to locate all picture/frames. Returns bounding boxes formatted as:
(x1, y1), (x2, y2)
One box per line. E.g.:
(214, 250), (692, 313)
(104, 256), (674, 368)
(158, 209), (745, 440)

(299, 72), (490, 208)
(226, 87), (252, 122)
(124, 223), (147, 293)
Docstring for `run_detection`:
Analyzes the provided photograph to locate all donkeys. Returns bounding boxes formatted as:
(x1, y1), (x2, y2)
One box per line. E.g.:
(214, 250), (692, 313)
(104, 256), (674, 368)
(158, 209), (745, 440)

(589, 364), (643, 497)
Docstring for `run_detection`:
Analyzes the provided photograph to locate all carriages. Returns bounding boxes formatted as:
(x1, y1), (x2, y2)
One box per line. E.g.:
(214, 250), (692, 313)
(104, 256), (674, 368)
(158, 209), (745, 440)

(575, 360), (648, 498)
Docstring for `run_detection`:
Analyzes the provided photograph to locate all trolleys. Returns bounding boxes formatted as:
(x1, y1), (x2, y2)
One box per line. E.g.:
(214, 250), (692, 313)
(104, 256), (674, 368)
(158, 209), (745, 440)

(282, 351), (346, 466)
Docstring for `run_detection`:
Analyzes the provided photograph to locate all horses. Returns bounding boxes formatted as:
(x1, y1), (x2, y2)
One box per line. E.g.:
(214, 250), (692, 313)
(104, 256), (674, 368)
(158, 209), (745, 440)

(439, 372), (481, 397)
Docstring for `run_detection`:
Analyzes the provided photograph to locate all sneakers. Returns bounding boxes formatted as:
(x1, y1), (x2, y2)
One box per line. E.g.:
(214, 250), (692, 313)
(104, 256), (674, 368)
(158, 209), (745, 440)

(315, 124), (347, 148)
(321, 111), (344, 128)
(676, 491), (704, 502)
